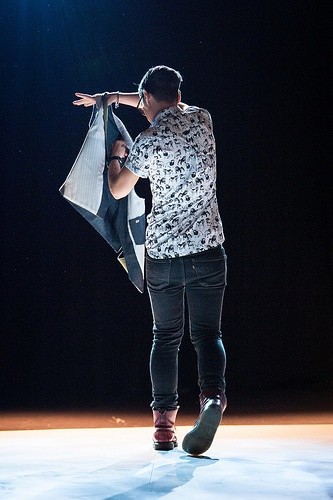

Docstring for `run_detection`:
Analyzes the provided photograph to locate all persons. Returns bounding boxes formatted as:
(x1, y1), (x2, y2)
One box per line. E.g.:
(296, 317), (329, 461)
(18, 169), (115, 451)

(73, 65), (227, 454)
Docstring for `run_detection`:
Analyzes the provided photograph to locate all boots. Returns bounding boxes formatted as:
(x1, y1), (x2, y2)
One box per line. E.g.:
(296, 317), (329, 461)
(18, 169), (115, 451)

(150, 407), (178, 450)
(181, 385), (228, 459)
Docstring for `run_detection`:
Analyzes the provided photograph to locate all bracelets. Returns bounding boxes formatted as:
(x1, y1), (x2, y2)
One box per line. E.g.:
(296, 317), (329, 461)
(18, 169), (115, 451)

(115, 91), (120, 108)
(107, 156), (122, 168)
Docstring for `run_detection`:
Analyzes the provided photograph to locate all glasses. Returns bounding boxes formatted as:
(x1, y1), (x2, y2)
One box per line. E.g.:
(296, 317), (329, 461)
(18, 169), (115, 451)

(135, 93), (148, 117)
